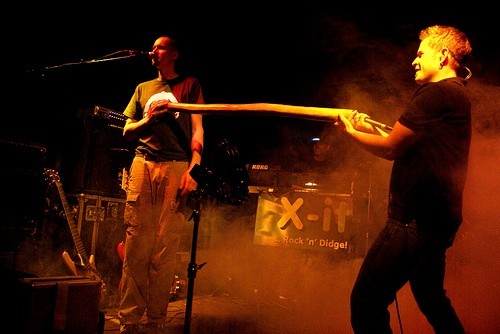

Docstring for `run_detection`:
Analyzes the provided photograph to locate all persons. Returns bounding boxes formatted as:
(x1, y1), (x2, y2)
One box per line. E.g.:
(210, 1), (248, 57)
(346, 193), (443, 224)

(333, 25), (472, 334)
(118, 36), (205, 334)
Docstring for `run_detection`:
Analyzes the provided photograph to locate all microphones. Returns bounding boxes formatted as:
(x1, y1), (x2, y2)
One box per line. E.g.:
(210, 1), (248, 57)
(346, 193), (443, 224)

(128, 50), (154, 60)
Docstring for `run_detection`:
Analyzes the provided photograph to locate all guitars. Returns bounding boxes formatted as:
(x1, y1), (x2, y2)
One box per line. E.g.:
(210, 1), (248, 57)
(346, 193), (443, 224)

(43, 168), (111, 310)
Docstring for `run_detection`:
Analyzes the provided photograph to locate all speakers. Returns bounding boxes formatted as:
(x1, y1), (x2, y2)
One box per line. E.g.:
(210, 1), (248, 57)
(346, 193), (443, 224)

(17, 278), (101, 334)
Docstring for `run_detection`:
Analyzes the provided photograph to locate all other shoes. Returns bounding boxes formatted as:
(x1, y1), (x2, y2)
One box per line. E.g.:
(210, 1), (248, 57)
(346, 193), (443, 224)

(120, 324), (140, 334)
(143, 322), (166, 334)
